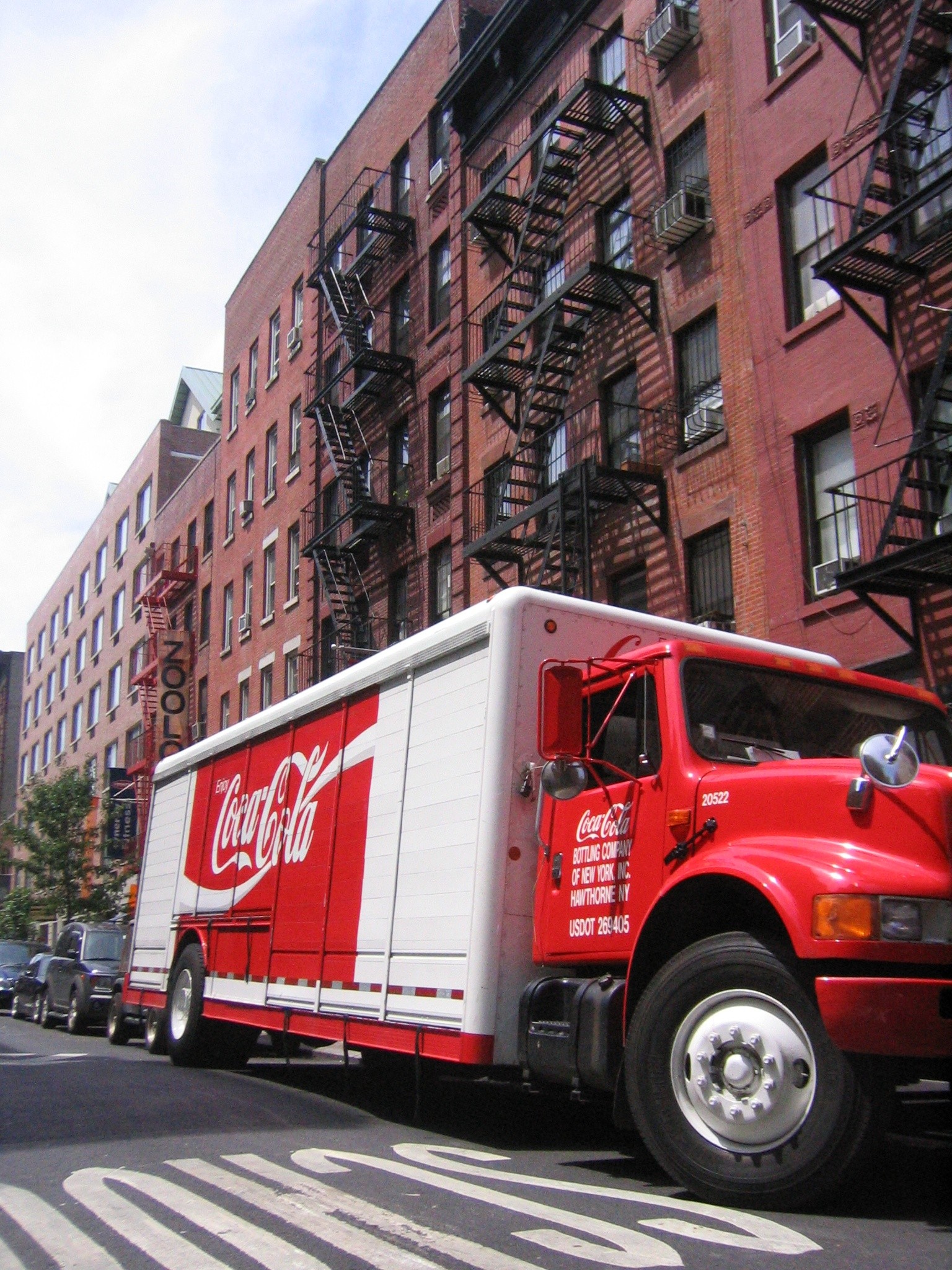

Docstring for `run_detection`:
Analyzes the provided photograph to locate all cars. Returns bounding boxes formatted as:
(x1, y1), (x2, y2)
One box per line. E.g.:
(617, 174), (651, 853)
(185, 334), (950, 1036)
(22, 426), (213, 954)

(9, 951), (54, 1025)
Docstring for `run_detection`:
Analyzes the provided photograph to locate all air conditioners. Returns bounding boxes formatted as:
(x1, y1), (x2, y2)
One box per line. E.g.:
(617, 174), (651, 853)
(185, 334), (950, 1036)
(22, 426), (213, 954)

(239, 500), (253, 519)
(245, 387), (257, 406)
(683, 405), (725, 446)
(654, 189), (710, 247)
(287, 326), (303, 349)
(436, 455), (450, 480)
(238, 613), (252, 633)
(774, 19), (817, 68)
(811, 557), (860, 597)
(644, 3), (699, 64)
(429, 158), (450, 186)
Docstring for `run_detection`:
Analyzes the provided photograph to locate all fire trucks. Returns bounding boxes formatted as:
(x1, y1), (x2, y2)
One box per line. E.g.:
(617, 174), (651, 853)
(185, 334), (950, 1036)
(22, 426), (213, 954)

(120, 584), (952, 1210)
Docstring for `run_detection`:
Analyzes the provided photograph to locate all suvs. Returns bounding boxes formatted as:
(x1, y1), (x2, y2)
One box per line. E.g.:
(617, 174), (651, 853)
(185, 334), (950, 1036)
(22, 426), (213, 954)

(0, 939), (52, 1009)
(39, 920), (129, 1034)
(106, 918), (176, 1055)
